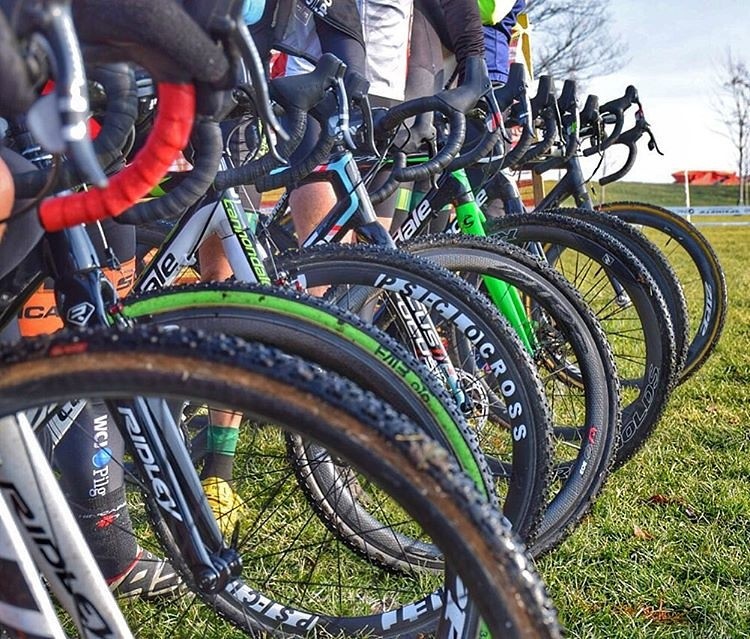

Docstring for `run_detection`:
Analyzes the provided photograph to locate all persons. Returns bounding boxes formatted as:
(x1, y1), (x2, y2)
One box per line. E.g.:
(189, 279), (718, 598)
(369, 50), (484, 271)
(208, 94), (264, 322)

(0, 157), (55, 639)
(104, 73), (259, 537)
(41, 73), (194, 597)
(367, 0), (540, 447)
(247, 0), (371, 424)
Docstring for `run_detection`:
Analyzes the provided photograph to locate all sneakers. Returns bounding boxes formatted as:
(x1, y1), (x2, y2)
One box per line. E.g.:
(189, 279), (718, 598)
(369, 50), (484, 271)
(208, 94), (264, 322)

(201, 477), (248, 536)
(104, 544), (190, 605)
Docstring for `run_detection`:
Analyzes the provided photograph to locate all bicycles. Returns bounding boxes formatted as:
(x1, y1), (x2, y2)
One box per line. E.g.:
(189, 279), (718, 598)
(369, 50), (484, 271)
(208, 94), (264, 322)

(0, 0), (727, 639)
